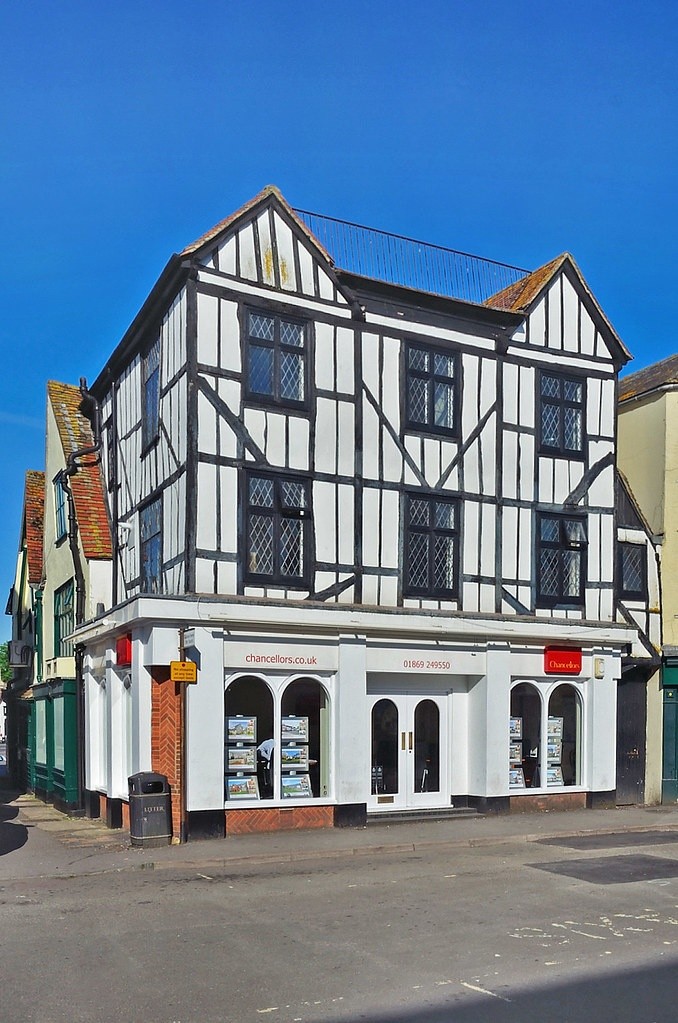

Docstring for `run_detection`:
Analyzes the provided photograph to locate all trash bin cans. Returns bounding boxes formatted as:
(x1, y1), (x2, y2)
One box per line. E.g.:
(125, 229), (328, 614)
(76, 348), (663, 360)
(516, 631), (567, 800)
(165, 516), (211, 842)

(128, 772), (172, 849)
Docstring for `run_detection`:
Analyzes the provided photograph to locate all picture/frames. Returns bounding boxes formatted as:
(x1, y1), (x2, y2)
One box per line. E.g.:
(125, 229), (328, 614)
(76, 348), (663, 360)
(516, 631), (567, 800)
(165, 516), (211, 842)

(546, 741), (563, 765)
(547, 717), (564, 739)
(281, 745), (309, 772)
(224, 746), (258, 773)
(281, 716), (310, 743)
(509, 768), (527, 789)
(509, 743), (523, 766)
(510, 717), (524, 740)
(281, 774), (313, 799)
(224, 775), (261, 801)
(224, 716), (258, 745)
(546, 766), (565, 787)
